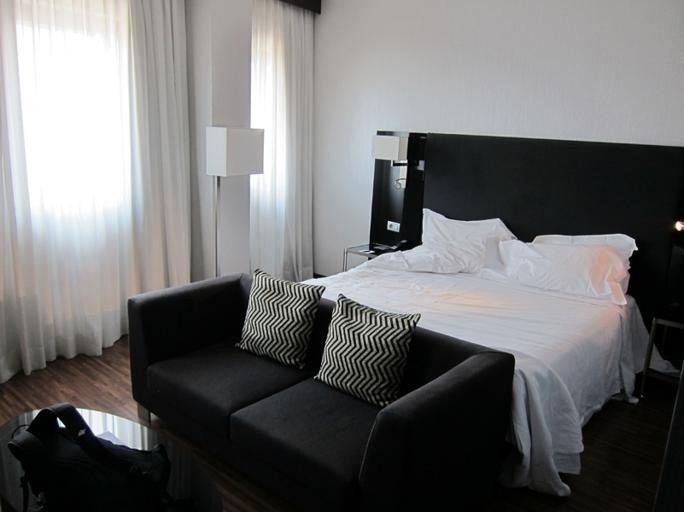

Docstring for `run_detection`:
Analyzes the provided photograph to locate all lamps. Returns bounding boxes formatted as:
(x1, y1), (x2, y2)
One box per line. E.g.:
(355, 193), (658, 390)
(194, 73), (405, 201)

(205, 126), (263, 278)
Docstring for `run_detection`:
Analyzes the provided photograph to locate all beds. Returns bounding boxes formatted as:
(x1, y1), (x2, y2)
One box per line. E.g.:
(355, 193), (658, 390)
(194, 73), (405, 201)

(294, 132), (683, 498)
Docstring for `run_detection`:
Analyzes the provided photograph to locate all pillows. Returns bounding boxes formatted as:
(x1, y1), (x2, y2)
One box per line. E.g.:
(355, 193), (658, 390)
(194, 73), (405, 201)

(417, 207), (638, 308)
(234, 267), (421, 409)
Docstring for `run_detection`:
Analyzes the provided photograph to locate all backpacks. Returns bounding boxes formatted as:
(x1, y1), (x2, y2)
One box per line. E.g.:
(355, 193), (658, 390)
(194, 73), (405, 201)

(9, 403), (170, 511)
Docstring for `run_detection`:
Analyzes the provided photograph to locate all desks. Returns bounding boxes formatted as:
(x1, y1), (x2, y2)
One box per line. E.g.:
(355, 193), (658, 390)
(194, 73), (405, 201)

(640, 317), (684, 399)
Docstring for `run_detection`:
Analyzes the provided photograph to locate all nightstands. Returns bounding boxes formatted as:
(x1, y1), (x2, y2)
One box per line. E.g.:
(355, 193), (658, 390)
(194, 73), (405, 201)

(344, 244), (394, 271)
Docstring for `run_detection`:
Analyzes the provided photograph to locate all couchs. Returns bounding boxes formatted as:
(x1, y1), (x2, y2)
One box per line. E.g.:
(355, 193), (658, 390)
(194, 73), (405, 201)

(129, 271), (513, 497)
